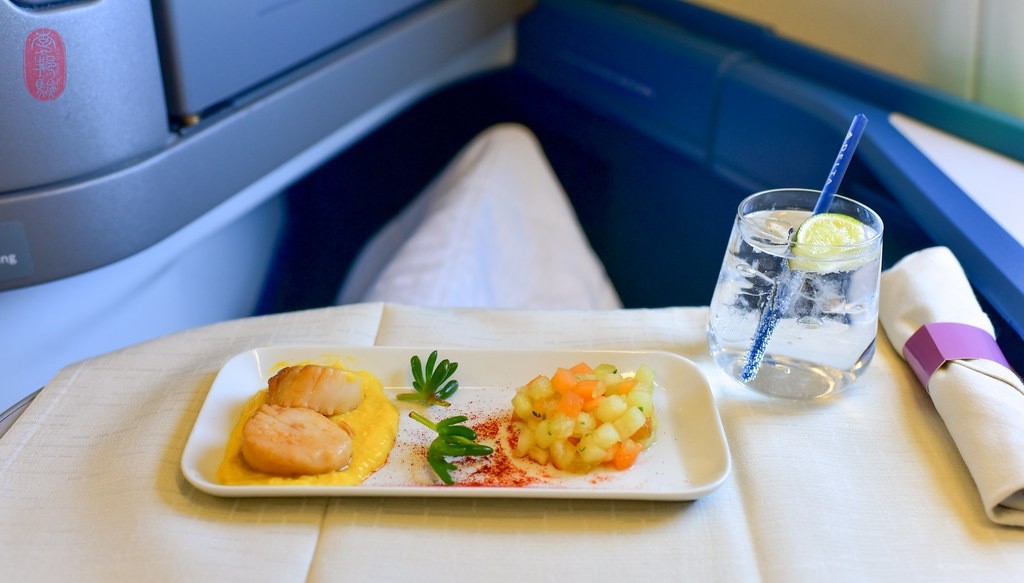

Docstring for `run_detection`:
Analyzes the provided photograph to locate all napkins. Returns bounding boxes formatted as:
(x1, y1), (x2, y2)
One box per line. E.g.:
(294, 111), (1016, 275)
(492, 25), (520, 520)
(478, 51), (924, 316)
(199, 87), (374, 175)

(875, 243), (1024, 527)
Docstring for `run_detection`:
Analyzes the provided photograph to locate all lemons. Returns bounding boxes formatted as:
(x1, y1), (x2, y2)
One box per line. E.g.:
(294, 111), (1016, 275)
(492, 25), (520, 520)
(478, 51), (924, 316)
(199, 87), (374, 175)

(788, 212), (880, 273)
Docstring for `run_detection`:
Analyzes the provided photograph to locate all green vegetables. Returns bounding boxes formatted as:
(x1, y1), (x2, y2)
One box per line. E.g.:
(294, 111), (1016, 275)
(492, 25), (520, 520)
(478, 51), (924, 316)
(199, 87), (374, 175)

(397, 349), (494, 485)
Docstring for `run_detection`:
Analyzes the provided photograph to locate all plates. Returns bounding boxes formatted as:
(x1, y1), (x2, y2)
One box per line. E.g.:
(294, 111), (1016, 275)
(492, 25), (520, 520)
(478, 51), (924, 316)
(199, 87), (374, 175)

(177, 343), (732, 503)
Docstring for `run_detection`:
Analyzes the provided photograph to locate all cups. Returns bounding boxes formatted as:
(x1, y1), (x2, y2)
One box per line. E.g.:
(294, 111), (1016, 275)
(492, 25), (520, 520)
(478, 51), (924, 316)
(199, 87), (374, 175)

(703, 188), (885, 399)
